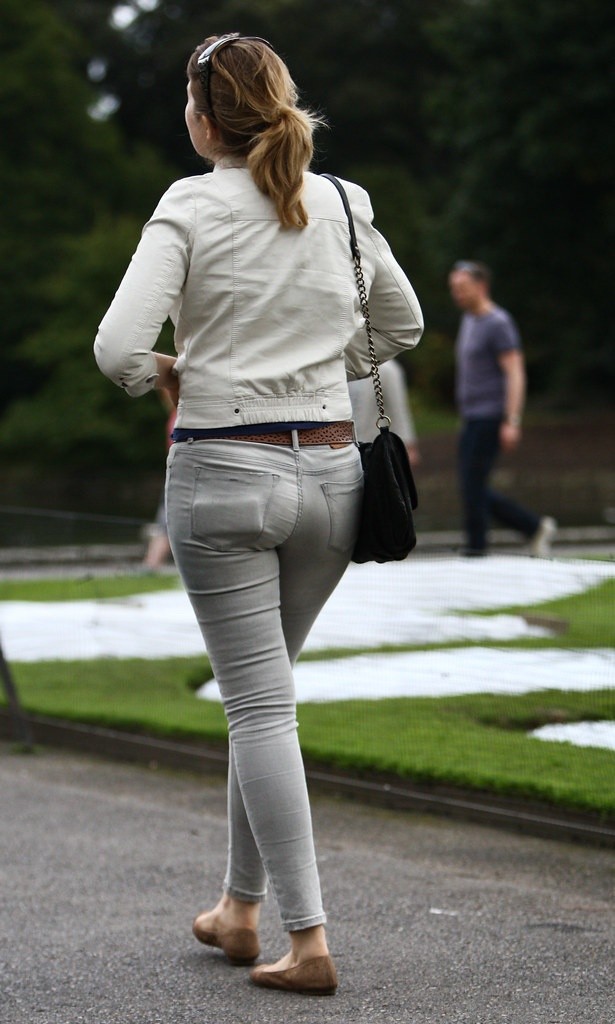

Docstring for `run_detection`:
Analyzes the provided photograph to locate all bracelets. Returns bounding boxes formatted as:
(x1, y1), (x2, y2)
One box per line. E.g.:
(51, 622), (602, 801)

(504, 415), (521, 426)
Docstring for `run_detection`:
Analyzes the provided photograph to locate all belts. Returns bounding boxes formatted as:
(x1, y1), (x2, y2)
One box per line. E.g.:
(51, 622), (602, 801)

(194, 420), (354, 446)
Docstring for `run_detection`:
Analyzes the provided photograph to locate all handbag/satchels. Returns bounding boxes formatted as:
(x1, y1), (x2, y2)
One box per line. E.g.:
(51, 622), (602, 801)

(343, 429), (416, 563)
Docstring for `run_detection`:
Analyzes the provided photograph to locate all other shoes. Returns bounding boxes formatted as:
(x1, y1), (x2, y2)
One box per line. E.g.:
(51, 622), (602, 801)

(192, 914), (261, 967)
(532, 516), (556, 558)
(452, 541), (488, 557)
(247, 954), (338, 998)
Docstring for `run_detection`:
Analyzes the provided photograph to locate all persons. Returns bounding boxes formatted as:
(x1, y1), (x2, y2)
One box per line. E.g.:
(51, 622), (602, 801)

(93, 33), (424, 994)
(448, 262), (555, 558)
(140, 385), (177, 565)
(349, 361), (417, 464)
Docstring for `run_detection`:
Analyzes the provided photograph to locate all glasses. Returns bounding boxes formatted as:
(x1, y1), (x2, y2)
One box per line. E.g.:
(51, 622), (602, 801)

(197, 34), (274, 117)
